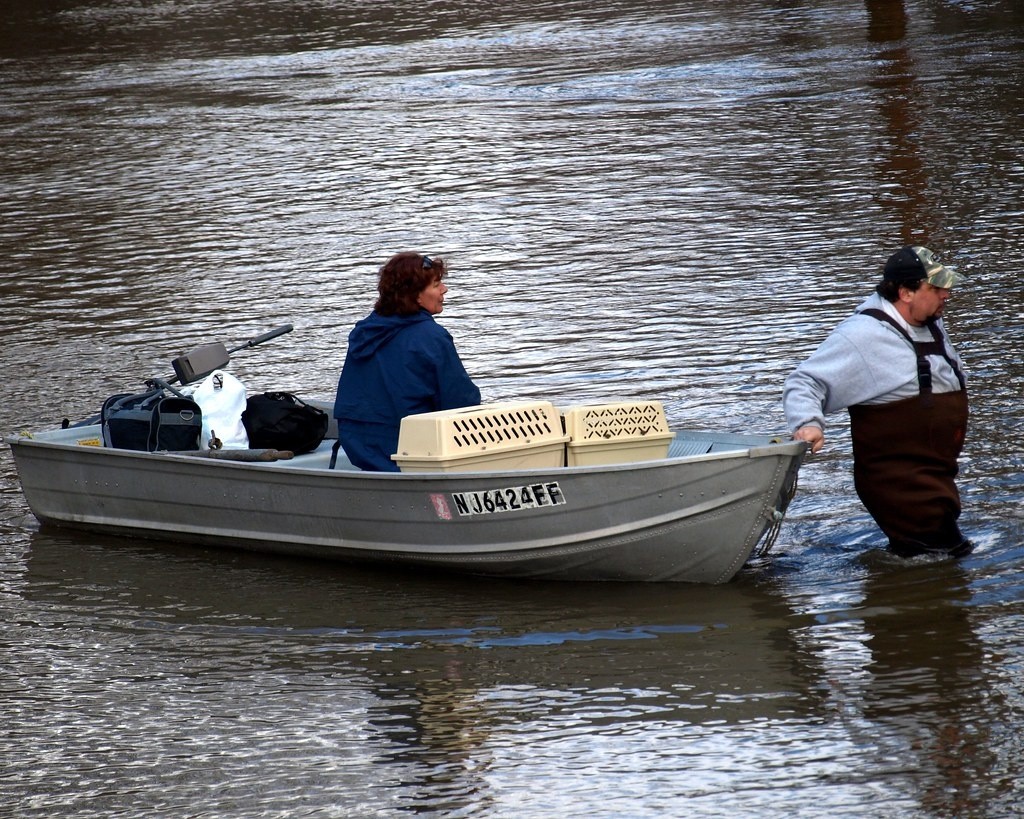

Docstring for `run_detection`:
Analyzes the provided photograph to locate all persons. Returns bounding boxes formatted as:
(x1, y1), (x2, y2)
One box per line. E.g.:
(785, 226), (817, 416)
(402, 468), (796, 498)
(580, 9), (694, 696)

(328, 253), (482, 472)
(783, 247), (975, 559)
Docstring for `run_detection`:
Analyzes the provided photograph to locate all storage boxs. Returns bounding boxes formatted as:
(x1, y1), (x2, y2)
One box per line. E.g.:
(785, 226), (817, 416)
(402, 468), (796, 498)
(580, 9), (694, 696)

(389, 398), (676, 471)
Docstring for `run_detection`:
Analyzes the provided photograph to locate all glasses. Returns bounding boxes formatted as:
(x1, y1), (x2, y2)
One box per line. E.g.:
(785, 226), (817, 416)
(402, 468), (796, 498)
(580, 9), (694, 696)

(421, 256), (432, 270)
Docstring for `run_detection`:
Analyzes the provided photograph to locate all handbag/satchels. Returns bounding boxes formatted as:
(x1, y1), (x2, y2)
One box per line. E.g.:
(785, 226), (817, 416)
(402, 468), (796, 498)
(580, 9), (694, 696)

(242, 391), (328, 456)
(101, 377), (203, 451)
(193, 370), (249, 450)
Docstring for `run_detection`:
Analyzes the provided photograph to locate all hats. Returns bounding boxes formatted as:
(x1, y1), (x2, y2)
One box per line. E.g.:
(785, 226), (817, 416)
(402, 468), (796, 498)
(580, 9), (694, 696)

(884, 246), (958, 288)
